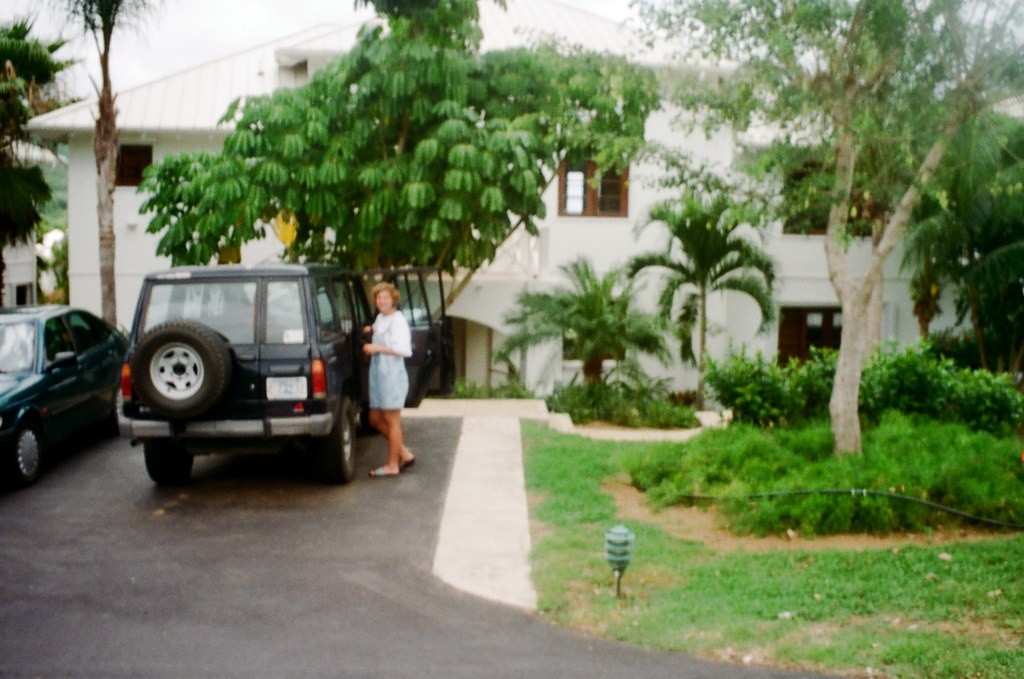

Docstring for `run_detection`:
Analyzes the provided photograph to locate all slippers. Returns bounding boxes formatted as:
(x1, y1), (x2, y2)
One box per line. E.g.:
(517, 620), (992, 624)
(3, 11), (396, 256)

(366, 465), (400, 477)
(399, 458), (416, 473)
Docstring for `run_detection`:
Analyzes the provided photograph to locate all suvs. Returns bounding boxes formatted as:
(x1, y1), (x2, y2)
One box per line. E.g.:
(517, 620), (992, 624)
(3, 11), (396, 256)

(115, 261), (441, 486)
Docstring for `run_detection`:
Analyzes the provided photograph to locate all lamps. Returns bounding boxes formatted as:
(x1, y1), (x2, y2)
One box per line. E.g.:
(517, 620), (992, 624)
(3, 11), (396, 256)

(604, 526), (635, 572)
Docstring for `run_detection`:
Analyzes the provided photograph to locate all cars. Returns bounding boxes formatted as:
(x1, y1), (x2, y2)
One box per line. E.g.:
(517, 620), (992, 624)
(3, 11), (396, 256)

(0, 304), (132, 490)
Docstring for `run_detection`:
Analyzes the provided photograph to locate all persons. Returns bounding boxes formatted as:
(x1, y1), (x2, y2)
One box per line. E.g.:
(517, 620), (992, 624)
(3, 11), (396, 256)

(360, 279), (415, 478)
